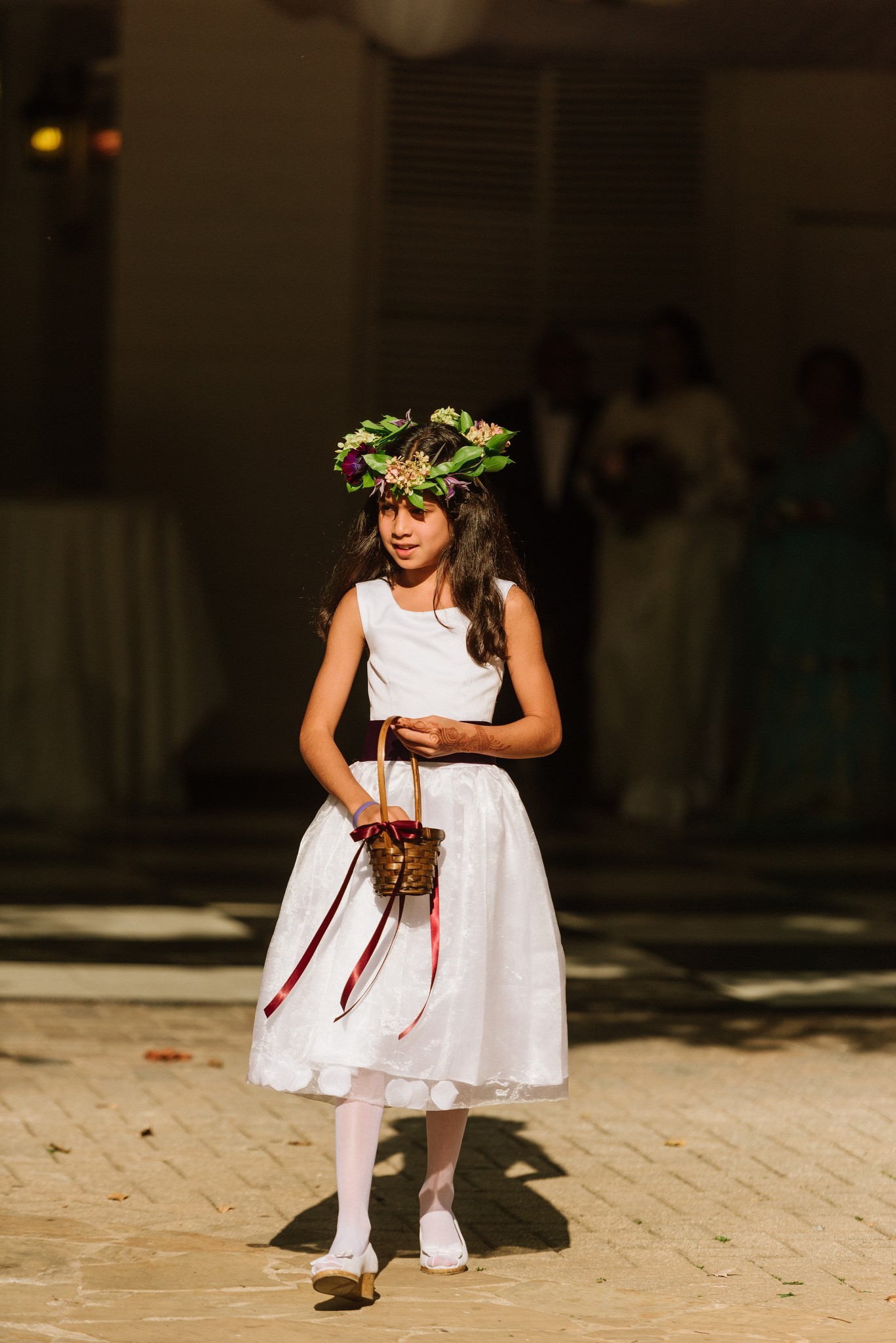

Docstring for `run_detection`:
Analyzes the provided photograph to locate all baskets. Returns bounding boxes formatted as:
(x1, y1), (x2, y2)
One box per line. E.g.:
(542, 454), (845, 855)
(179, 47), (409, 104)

(363, 716), (446, 897)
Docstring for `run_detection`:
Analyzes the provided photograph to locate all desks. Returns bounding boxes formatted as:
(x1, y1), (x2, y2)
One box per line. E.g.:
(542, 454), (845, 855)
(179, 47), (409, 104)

(0, 488), (237, 824)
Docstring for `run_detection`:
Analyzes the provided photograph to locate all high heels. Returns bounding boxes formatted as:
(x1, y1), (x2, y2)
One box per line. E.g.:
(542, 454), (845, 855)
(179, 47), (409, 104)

(311, 1243), (378, 1300)
(418, 1212), (468, 1276)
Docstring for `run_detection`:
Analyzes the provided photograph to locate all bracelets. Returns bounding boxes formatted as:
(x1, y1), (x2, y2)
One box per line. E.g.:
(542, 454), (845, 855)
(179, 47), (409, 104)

(353, 801), (380, 830)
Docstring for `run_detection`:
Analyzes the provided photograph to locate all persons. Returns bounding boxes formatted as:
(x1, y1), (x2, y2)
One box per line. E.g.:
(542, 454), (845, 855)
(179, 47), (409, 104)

(576, 304), (747, 835)
(472, 326), (612, 834)
(249, 407), (567, 1304)
(716, 343), (895, 844)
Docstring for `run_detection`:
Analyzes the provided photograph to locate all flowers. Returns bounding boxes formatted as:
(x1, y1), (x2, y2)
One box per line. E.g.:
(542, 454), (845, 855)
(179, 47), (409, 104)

(324, 402), (519, 518)
